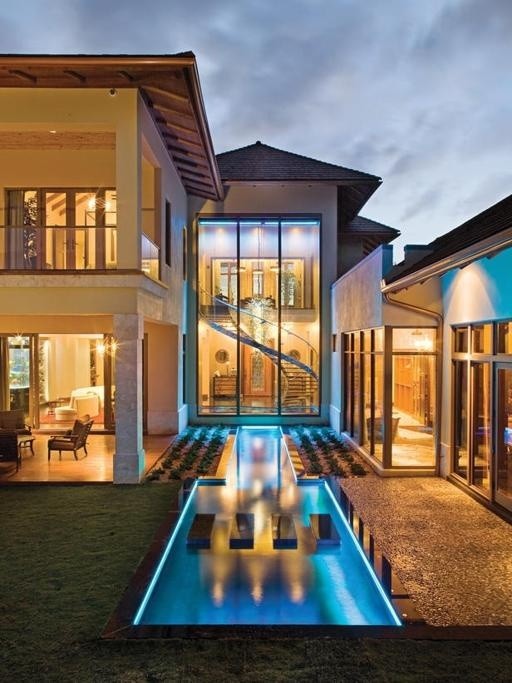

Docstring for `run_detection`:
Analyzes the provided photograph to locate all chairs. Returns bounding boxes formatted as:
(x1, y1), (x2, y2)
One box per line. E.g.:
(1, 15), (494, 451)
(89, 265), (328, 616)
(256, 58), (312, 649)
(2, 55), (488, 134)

(48, 414), (94, 461)
(1, 409), (32, 472)
